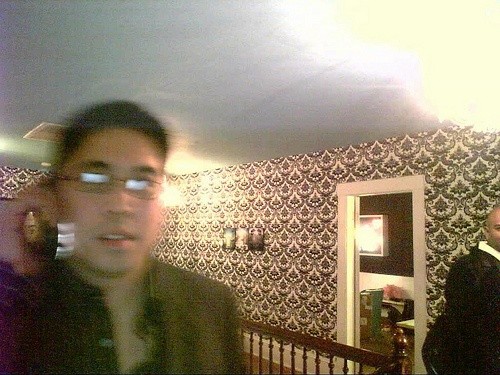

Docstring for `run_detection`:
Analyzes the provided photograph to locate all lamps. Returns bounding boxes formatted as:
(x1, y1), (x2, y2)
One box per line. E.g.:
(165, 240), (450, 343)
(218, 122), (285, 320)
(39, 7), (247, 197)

(222, 226), (265, 254)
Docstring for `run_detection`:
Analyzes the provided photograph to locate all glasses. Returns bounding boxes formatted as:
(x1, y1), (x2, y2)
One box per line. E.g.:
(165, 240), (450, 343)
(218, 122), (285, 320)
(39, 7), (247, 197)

(56, 173), (164, 201)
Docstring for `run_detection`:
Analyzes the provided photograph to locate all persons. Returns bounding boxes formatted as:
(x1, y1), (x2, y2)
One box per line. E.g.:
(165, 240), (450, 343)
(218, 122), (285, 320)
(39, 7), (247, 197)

(422, 205), (500, 374)
(1, 100), (248, 375)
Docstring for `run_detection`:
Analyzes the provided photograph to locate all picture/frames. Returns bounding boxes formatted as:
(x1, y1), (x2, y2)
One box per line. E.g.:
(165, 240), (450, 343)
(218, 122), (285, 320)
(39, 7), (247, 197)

(359, 215), (390, 257)
